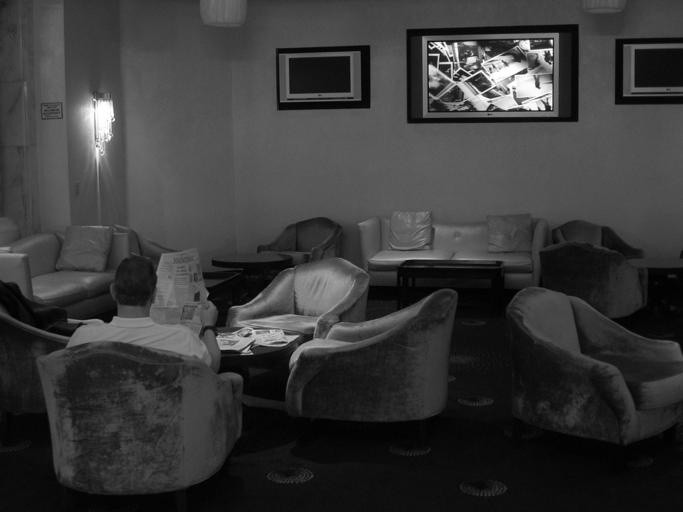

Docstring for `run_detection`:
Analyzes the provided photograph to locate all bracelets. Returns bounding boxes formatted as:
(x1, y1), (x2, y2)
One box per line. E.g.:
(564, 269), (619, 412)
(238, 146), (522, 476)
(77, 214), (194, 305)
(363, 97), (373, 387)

(202, 325), (218, 337)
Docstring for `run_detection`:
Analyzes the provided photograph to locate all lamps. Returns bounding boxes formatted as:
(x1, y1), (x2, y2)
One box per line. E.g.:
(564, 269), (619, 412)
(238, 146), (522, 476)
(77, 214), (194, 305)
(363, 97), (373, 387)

(81, 86), (116, 226)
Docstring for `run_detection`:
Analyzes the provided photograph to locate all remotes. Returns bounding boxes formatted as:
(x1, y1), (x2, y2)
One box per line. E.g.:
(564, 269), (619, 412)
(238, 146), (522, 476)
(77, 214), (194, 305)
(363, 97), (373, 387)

(242, 330), (249, 336)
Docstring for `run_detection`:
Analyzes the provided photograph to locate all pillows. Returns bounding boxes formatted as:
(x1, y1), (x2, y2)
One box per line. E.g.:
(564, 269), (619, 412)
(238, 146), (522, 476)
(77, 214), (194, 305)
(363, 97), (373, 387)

(55, 223), (115, 276)
(484, 211), (534, 255)
(390, 208), (433, 249)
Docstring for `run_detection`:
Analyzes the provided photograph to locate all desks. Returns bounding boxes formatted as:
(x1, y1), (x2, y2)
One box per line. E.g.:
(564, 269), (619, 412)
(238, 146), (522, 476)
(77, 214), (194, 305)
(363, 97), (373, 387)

(211, 252), (293, 272)
(149, 311), (306, 373)
(621, 253), (683, 311)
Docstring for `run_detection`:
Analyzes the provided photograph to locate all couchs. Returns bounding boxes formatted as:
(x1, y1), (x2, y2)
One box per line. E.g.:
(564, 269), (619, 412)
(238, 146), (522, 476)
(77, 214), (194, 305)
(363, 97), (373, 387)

(356, 209), (549, 292)
(538, 218), (648, 261)
(114, 218), (185, 281)
(505, 284), (682, 476)
(256, 216), (343, 258)
(225, 256), (373, 340)
(33, 338), (242, 495)
(539, 242), (647, 321)
(0, 306), (71, 415)
(0, 221), (134, 318)
(284, 287), (457, 473)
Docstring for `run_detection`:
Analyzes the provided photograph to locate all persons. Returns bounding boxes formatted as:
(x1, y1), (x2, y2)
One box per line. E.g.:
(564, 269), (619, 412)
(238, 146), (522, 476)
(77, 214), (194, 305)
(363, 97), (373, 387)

(66, 256), (221, 375)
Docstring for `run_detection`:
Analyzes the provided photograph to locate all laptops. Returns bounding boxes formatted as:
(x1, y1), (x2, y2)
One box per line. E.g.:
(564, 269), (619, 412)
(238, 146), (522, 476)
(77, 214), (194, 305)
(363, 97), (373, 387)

(630, 45), (683, 96)
(282, 54), (358, 102)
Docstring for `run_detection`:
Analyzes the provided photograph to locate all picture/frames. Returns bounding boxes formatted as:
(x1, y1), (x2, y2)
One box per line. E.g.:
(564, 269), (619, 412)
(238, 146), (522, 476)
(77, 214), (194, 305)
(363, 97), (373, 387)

(404, 23), (581, 123)
(276, 45), (370, 111)
(614, 36), (682, 105)
